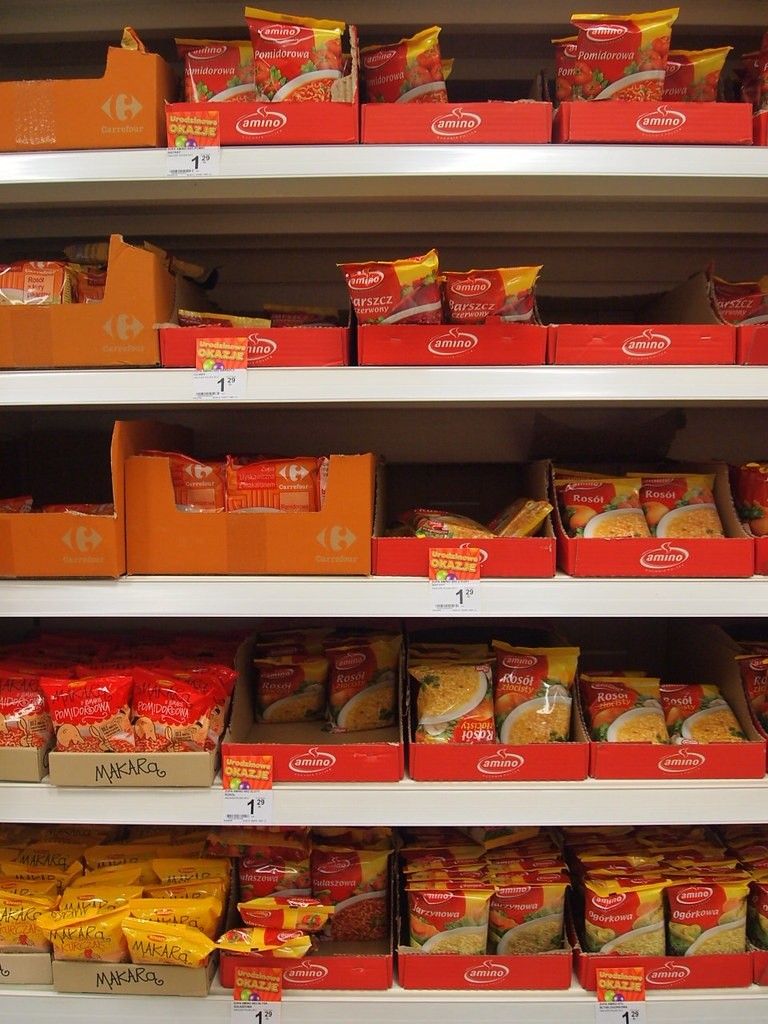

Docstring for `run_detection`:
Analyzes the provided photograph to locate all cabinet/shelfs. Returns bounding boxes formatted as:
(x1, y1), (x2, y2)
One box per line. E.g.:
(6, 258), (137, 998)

(0, 0), (768, 1024)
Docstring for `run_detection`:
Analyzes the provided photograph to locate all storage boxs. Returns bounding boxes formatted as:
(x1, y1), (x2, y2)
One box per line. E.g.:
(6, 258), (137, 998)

(220, 626), (405, 784)
(533, 271), (737, 365)
(161, 25), (359, 148)
(0, 234), (175, 371)
(0, 831), (768, 998)
(0, 417), (159, 580)
(371, 454), (558, 579)
(49, 745), (220, 788)
(543, 48), (754, 146)
(125, 451), (377, 577)
(0, 740), (55, 783)
(159, 270), (356, 368)
(399, 618), (591, 782)
(359, 71), (554, 144)
(357, 313), (548, 366)
(549, 459), (756, 579)
(704, 622), (768, 774)
(688, 260), (767, 367)
(574, 633), (768, 780)
(0, 45), (184, 153)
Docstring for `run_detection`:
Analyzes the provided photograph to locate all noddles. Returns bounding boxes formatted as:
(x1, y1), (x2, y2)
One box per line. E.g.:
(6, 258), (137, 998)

(260, 504), (768, 956)
